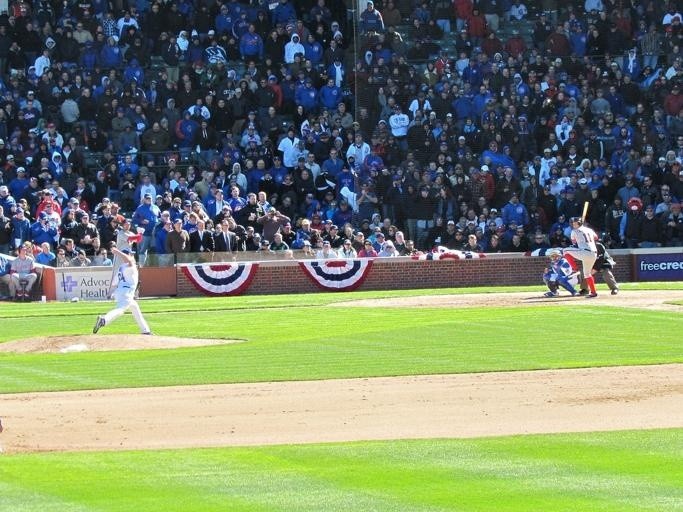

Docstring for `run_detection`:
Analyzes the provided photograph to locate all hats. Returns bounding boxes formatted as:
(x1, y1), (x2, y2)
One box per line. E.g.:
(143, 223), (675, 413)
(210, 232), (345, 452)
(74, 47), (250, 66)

(121, 248), (135, 255)
(0, 1), (683, 251)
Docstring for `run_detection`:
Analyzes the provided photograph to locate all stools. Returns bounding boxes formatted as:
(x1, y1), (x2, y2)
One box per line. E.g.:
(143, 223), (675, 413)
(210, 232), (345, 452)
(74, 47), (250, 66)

(0, 281), (11, 300)
(18, 281), (29, 302)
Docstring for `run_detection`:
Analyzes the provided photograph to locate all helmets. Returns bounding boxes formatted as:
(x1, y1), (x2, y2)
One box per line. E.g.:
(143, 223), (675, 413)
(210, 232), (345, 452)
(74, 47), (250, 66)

(572, 216), (582, 226)
(548, 249), (562, 265)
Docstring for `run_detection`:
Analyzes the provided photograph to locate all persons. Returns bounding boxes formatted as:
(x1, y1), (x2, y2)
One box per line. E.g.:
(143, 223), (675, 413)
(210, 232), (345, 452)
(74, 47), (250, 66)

(92, 244), (154, 335)
(0, 0), (683, 302)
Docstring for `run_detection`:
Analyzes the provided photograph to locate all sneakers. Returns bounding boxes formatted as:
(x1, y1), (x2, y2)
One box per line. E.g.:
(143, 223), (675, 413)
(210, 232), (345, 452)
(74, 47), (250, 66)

(572, 290), (581, 296)
(93, 316), (105, 334)
(544, 291), (559, 297)
(567, 269), (580, 279)
(585, 293), (597, 298)
(23, 289), (30, 297)
(580, 289), (589, 294)
(611, 288), (618, 295)
(17, 289), (22, 297)
(9, 296), (17, 301)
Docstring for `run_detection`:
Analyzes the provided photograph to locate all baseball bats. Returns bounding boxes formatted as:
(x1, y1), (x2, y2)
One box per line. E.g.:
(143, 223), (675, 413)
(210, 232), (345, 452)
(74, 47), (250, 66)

(581, 202), (589, 225)
(34, 266), (44, 299)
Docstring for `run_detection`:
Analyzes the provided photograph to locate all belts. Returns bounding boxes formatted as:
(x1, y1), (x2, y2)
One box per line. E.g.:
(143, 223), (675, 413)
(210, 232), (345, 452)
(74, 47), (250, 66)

(585, 249), (597, 253)
